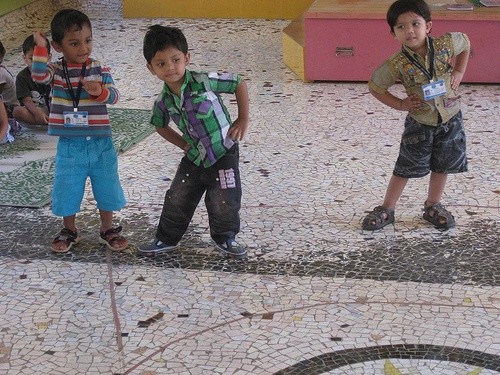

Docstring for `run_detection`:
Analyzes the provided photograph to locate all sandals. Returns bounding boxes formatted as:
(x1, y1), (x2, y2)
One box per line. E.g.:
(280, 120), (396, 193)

(423, 200), (455, 227)
(362, 205), (395, 231)
(50, 228), (79, 252)
(99, 226), (128, 251)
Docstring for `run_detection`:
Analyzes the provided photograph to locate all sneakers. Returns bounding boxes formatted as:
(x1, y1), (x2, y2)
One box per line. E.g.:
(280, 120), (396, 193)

(138, 238), (180, 252)
(211, 237), (247, 255)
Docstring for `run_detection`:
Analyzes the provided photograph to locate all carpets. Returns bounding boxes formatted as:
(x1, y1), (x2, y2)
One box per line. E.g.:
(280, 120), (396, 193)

(0, 107), (157, 208)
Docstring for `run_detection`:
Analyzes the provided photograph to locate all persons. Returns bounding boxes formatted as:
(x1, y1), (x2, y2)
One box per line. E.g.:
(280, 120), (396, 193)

(31, 9), (128, 253)
(137, 25), (249, 256)
(425, 90), (429, 95)
(362, 0), (470, 231)
(0, 34), (53, 144)
(66, 118), (70, 123)
